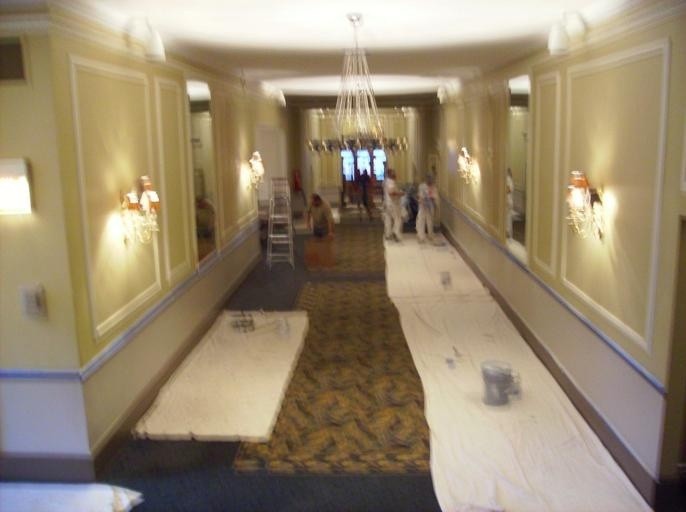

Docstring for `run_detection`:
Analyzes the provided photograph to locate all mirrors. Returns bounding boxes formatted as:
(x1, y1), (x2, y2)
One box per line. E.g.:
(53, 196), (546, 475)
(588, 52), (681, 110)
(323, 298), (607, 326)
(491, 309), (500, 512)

(178, 69), (220, 268)
(501, 69), (532, 266)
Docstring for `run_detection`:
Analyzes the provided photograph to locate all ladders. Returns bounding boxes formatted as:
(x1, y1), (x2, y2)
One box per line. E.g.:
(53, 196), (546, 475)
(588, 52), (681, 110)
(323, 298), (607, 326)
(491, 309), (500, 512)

(266, 176), (296, 273)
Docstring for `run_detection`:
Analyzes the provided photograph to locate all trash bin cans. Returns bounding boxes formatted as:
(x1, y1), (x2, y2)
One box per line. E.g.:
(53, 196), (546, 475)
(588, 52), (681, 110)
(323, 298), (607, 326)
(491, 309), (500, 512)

(483, 368), (513, 405)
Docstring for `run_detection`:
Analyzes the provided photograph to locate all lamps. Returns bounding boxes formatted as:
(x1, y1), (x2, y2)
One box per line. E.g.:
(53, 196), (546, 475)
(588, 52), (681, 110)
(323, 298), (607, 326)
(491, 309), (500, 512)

(453, 144), (477, 182)
(305, 13), (414, 152)
(120, 174), (157, 241)
(0, 156), (32, 217)
(559, 169), (602, 241)
(239, 151), (266, 192)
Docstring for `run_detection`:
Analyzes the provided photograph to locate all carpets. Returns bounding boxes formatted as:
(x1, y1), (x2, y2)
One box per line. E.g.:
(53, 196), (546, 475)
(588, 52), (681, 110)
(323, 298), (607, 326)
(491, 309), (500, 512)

(131, 306), (310, 445)
(1, 482), (146, 511)
(382, 230), (655, 510)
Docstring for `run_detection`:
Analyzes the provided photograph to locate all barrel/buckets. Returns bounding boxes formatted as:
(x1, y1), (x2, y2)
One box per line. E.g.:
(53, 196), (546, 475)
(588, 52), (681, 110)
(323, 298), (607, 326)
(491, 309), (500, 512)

(481, 362), (512, 405)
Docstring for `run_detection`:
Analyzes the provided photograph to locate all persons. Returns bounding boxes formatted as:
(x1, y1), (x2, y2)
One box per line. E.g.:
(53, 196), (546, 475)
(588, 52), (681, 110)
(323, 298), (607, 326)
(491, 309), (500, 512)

(342, 169), (438, 243)
(307, 194), (335, 241)
(198, 199), (215, 244)
(504, 168), (514, 241)
